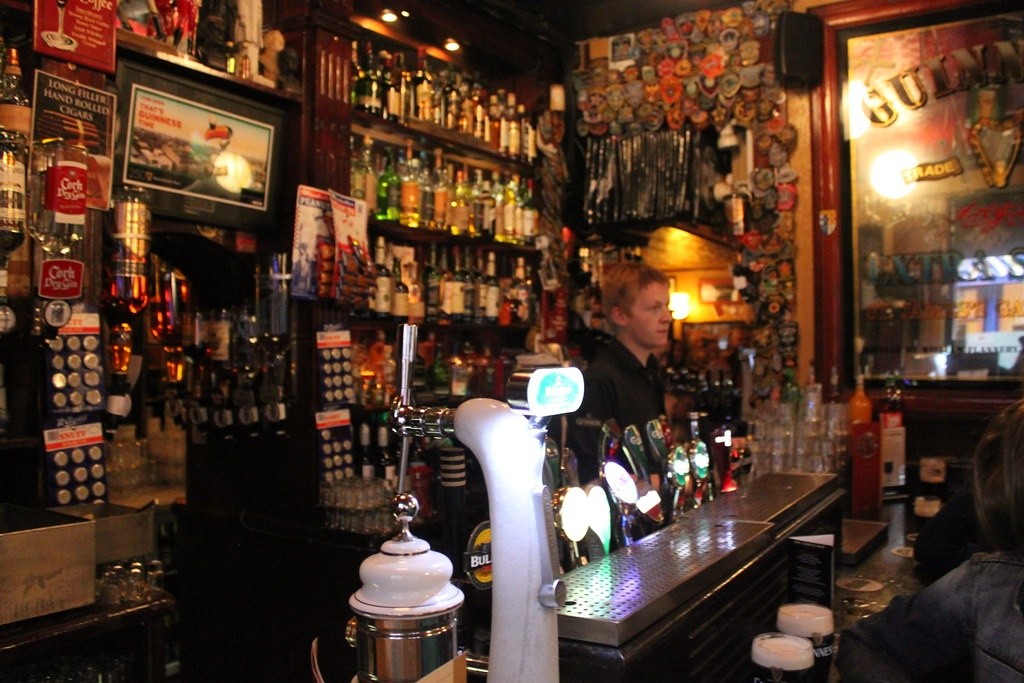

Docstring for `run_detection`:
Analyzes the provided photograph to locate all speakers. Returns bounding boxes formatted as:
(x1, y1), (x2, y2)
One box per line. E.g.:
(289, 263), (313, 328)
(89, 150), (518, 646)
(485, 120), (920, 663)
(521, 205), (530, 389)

(773, 11), (825, 88)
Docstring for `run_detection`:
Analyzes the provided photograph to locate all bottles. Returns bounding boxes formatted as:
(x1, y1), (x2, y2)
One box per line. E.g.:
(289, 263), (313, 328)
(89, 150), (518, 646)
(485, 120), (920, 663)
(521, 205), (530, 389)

(354, 423), (375, 481)
(349, 50), (550, 245)
(352, 246), (541, 324)
(849, 375), (872, 421)
(781, 370), (800, 410)
(880, 377), (904, 428)
(377, 427), (397, 479)
(409, 436), (426, 468)
(413, 341), (511, 398)
(663, 367), (731, 413)
(0, 47), (31, 105)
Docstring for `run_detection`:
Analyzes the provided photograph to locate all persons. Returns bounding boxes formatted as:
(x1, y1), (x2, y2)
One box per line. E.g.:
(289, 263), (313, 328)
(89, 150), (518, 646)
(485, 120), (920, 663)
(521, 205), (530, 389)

(835, 396), (1024, 682)
(543, 262), (695, 518)
(914, 501), (985, 571)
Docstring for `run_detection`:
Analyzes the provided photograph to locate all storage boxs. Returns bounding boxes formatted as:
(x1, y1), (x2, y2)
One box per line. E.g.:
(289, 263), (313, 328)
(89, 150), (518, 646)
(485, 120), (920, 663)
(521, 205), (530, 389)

(59, 502), (155, 564)
(0, 502), (97, 627)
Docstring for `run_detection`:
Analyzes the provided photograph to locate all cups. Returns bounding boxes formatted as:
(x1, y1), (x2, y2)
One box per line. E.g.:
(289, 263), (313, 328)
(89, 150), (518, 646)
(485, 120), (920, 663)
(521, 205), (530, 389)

(753, 632), (816, 683)
(441, 447), (466, 487)
(775, 602), (833, 683)
(746, 384), (849, 475)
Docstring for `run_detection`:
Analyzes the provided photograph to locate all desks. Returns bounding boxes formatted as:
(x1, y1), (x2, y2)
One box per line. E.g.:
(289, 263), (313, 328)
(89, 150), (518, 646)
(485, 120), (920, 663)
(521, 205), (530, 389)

(0, 585), (177, 683)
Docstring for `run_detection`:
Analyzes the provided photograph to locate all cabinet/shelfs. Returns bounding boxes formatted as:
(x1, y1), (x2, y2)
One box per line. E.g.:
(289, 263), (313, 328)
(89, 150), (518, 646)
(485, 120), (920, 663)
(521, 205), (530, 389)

(264, 18), (542, 683)
